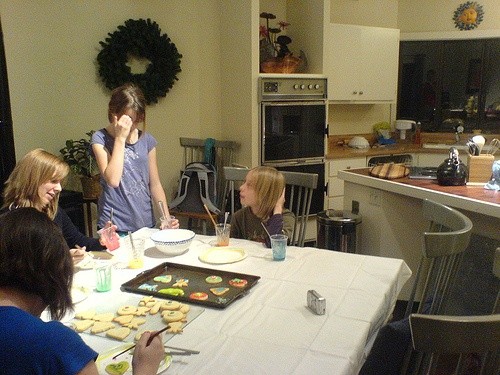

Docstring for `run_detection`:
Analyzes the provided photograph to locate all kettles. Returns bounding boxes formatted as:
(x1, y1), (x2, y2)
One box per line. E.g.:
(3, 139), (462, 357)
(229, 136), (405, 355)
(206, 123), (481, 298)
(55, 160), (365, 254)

(437, 147), (469, 186)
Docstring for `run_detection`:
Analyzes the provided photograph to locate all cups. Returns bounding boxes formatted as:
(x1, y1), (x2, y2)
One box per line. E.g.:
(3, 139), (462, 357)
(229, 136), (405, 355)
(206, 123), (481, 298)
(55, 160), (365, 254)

(269, 234), (288, 261)
(93, 262), (112, 292)
(62, 321), (78, 333)
(159, 215), (175, 231)
(124, 237), (146, 269)
(214, 223), (231, 247)
(97, 225), (121, 252)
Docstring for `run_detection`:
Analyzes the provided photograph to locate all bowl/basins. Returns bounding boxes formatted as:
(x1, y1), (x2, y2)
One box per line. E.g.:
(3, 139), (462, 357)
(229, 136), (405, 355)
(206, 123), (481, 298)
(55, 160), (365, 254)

(348, 136), (371, 148)
(150, 229), (196, 257)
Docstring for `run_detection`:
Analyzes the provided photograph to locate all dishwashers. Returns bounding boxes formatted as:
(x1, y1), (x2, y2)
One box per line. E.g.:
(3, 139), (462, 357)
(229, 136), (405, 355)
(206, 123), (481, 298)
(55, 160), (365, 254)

(366, 153), (416, 168)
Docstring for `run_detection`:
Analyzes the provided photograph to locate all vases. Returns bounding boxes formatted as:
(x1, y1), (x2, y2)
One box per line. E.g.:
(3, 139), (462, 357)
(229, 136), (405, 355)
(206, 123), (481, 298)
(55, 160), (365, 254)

(260, 56), (304, 74)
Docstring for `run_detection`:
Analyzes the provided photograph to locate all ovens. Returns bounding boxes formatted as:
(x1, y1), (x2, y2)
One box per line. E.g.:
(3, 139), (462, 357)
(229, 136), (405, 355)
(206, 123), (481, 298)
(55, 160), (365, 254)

(258, 74), (329, 218)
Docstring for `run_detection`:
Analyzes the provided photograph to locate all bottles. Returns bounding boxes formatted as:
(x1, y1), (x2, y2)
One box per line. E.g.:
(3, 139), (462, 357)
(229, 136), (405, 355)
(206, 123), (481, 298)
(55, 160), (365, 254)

(415, 121), (422, 144)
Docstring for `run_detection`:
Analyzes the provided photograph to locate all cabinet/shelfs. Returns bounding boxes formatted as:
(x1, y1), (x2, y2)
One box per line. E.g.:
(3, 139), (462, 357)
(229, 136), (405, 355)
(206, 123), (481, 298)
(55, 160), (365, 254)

(219, 0), (400, 244)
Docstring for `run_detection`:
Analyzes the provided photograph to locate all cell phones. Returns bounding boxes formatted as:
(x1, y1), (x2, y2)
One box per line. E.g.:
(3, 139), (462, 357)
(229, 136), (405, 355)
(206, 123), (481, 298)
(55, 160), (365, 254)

(306, 290), (326, 315)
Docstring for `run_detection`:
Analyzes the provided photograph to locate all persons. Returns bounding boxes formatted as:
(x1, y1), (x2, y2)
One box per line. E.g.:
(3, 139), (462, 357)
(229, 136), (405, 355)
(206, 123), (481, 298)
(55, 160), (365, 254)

(91, 87), (180, 239)
(0, 207), (165, 375)
(230, 166), (295, 248)
(0, 147), (120, 265)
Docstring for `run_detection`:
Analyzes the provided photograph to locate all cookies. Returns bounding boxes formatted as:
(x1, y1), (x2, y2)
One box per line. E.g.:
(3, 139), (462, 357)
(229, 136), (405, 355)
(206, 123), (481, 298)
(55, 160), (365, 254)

(138, 275), (248, 301)
(70, 296), (190, 342)
(105, 361), (129, 375)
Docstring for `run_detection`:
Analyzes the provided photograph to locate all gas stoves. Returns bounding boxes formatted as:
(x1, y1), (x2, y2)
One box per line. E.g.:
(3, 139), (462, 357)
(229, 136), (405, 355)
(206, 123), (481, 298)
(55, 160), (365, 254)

(409, 165), (439, 179)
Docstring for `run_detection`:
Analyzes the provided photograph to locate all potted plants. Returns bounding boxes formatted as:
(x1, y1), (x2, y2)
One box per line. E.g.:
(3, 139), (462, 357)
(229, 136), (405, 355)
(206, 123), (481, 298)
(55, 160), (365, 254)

(60, 130), (102, 198)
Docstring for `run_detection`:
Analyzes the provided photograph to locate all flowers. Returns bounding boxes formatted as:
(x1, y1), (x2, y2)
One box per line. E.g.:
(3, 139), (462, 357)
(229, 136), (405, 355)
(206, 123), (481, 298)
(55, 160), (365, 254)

(259, 12), (308, 65)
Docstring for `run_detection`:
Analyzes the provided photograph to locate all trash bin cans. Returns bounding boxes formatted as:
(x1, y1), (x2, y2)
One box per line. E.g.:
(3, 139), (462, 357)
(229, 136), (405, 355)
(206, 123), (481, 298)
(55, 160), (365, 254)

(316, 209), (362, 255)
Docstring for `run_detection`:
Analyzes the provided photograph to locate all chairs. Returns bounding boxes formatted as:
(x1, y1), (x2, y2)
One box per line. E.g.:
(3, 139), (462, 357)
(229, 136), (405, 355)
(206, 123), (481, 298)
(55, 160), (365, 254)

(409, 247), (500, 374)
(169, 137), (241, 235)
(224, 166), (318, 247)
(359, 198), (473, 374)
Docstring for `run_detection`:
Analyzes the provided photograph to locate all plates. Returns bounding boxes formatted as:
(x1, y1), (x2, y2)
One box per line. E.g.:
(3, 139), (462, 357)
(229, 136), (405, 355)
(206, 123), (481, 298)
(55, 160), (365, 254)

(95, 342), (172, 375)
(79, 251), (114, 270)
(70, 283), (89, 306)
(198, 246), (247, 264)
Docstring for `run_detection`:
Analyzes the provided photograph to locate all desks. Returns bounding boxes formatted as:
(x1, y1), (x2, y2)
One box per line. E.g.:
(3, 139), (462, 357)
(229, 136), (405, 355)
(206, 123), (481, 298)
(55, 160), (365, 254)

(40, 227), (412, 375)
(77, 198), (98, 237)
